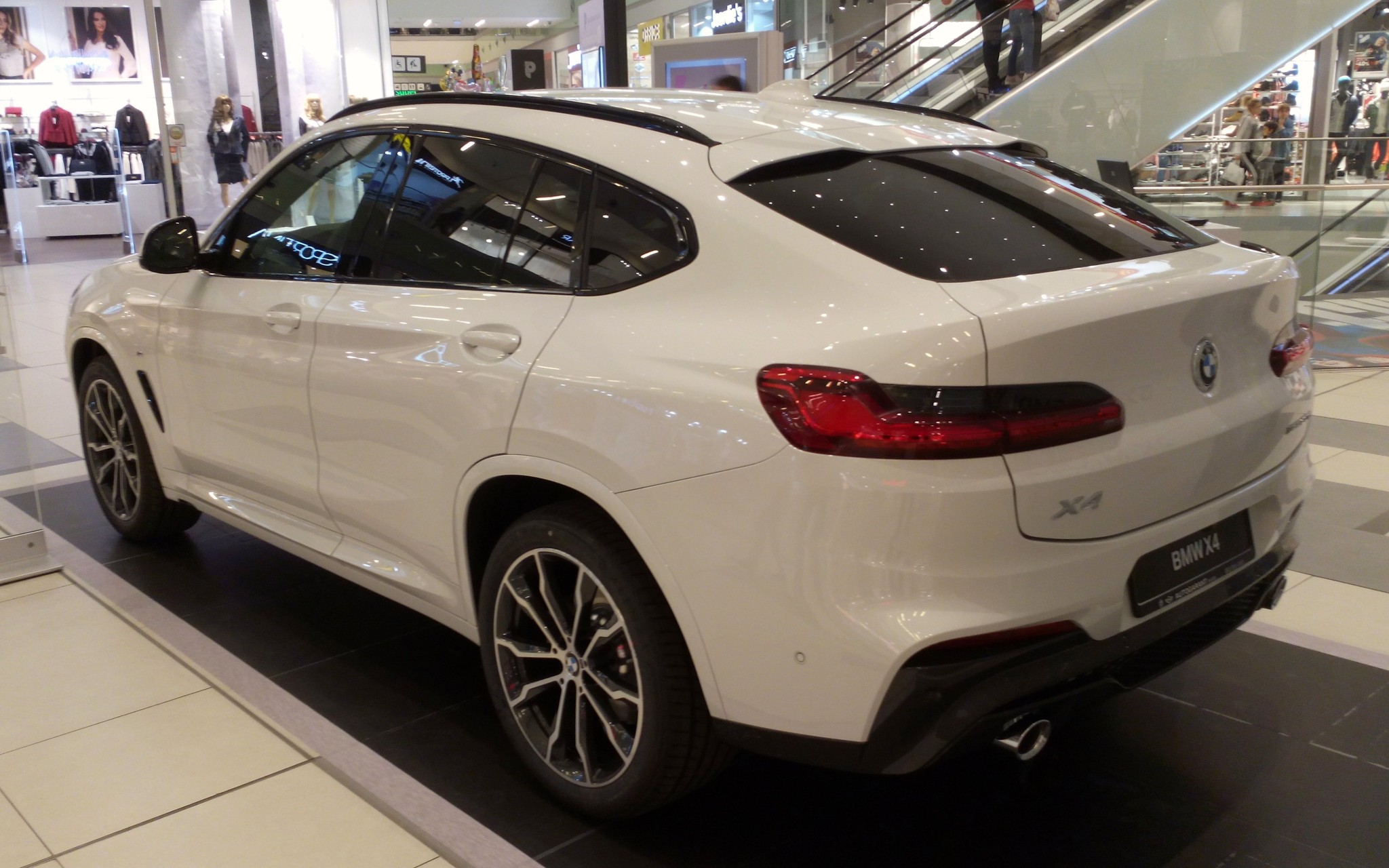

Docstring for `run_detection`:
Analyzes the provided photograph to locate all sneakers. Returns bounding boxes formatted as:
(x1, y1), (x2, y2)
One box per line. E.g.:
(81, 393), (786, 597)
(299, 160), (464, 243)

(1139, 130), (1208, 184)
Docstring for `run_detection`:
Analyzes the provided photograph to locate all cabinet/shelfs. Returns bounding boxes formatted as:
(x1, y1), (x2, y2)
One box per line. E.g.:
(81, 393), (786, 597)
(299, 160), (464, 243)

(1136, 75), (1306, 187)
(2, 147), (167, 240)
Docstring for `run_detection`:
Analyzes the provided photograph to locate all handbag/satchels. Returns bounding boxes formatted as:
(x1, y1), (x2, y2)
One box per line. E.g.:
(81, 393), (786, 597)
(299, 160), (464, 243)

(1045, 0), (1060, 21)
(1219, 159), (1245, 185)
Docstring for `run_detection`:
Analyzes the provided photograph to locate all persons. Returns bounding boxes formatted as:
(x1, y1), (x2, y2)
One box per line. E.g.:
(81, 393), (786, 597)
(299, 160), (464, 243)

(207, 97), (250, 208)
(75, 8), (137, 78)
(0, 11), (45, 80)
(1224, 95), (1294, 209)
(298, 95), (341, 226)
(1362, 78), (1389, 182)
(975, 0), (1042, 97)
(1356, 36), (1389, 71)
(1324, 74), (1358, 183)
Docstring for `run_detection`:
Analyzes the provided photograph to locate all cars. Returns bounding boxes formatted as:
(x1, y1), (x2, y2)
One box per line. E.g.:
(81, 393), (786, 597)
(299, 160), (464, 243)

(62, 90), (1318, 831)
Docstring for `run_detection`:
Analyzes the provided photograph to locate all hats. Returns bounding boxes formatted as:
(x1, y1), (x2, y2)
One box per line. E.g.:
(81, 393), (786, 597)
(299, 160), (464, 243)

(1379, 82), (1389, 92)
(1339, 76), (1351, 85)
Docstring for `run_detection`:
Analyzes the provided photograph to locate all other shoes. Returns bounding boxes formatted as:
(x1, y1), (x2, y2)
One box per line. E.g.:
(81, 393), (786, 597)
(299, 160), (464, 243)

(1212, 99), (1243, 166)
(1372, 161), (1380, 176)
(1224, 200), (1240, 208)
(1022, 70), (1035, 81)
(1366, 178), (1375, 182)
(989, 87), (1010, 96)
(1275, 198), (1281, 203)
(1250, 199), (1267, 207)
(1257, 200), (1276, 206)
(1253, 63), (1299, 106)
(1384, 170), (1389, 180)
(1327, 163), (1338, 180)
(1004, 75), (1022, 84)
(1284, 125), (1303, 183)
